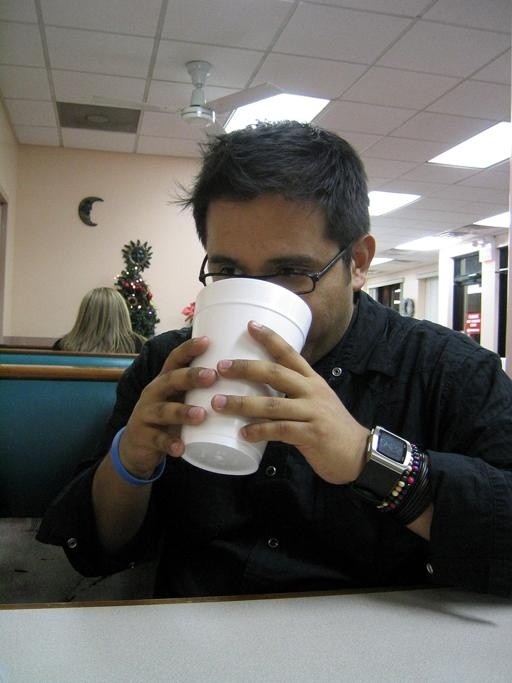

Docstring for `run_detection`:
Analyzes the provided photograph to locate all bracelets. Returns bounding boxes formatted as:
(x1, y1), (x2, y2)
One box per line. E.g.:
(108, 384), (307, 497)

(375, 442), (433, 528)
(110, 424), (168, 486)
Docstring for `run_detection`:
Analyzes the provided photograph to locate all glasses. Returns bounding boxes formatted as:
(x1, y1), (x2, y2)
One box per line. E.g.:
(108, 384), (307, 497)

(199, 239), (355, 295)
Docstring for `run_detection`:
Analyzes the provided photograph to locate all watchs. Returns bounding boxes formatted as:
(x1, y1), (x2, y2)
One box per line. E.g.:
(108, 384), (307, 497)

(350, 423), (412, 507)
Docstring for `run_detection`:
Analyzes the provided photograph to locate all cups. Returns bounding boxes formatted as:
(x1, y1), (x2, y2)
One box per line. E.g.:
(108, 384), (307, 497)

(177, 276), (315, 478)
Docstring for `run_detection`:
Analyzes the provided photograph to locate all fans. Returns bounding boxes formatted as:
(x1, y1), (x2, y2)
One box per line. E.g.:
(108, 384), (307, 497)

(92, 59), (284, 140)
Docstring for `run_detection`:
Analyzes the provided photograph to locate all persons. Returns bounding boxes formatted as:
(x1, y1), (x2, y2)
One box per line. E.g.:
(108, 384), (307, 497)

(32, 119), (512, 599)
(49, 285), (149, 355)
